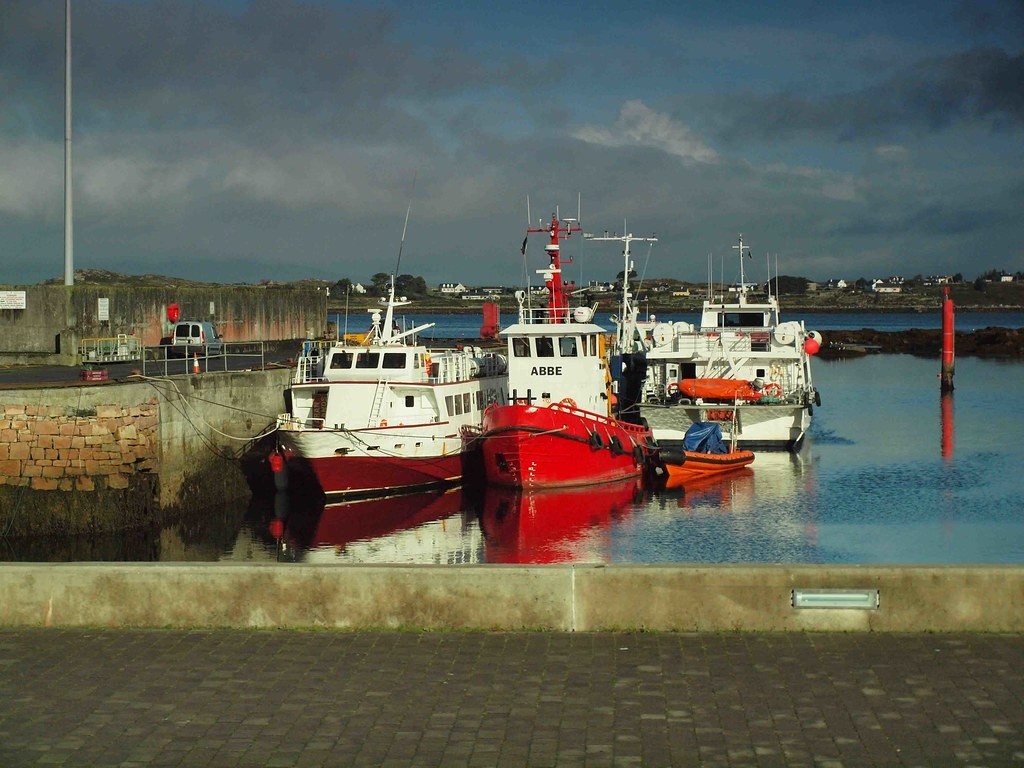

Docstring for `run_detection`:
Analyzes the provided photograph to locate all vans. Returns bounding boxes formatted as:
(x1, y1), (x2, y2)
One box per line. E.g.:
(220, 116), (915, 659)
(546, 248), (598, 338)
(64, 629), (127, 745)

(168, 321), (223, 356)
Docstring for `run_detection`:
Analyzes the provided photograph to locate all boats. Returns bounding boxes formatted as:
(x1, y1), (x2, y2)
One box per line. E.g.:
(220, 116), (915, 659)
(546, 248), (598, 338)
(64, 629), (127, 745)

(580, 205), (822, 447)
(481, 205), (664, 487)
(253, 487), (484, 566)
(652, 392), (755, 490)
(268, 170), (527, 504)
(677, 379), (767, 402)
(478, 482), (653, 566)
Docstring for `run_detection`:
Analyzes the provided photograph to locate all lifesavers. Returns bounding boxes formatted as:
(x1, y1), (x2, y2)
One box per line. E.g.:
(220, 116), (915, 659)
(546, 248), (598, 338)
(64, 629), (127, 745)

(557, 398), (578, 414)
(765, 383), (781, 399)
(380, 418), (387, 426)
(667, 383), (681, 397)
(511, 399), (532, 406)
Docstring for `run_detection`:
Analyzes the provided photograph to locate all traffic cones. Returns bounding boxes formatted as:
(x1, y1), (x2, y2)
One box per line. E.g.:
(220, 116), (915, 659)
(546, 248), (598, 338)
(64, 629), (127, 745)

(193, 352), (201, 373)
